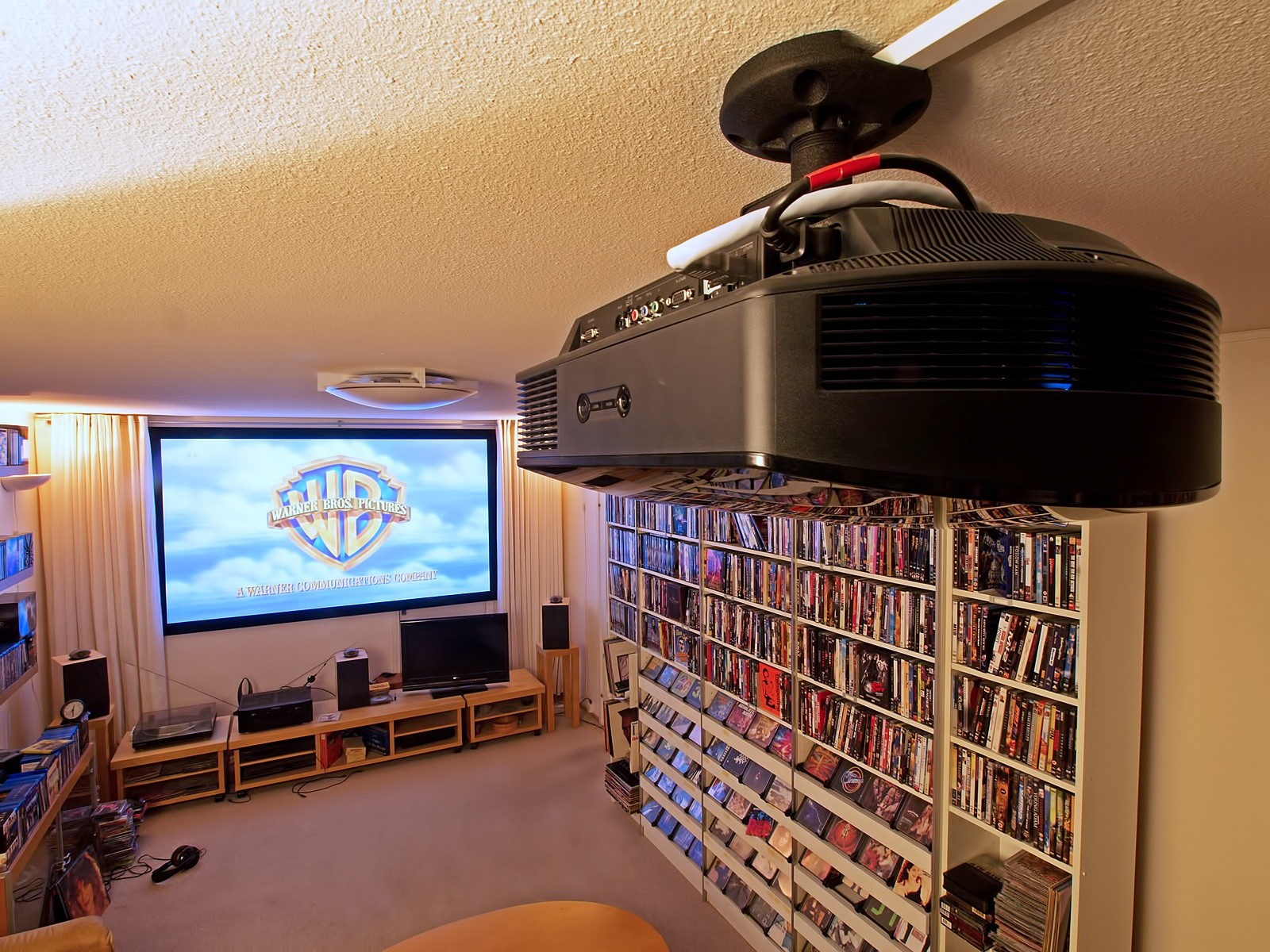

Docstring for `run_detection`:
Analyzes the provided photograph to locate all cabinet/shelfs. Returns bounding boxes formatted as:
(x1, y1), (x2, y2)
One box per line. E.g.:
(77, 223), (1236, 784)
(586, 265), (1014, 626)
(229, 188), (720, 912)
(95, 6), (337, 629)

(601, 492), (640, 816)
(702, 507), (794, 952)
(462, 667), (546, 750)
(793, 521), (937, 952)
(109, 713), (231, 812)
(0, 740), (101, 938)
(637, 500), (706, 898)
(227, 687), (466, 791)
(934, 522), (1150, 952)
(0, 528), (41, 712)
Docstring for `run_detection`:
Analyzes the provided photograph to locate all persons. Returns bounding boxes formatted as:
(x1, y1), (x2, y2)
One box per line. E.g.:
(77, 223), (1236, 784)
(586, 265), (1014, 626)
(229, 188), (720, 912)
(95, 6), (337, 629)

(859, 777), (934, 908)
(761, 668), (775, 709)
(651, 618), (657, 635)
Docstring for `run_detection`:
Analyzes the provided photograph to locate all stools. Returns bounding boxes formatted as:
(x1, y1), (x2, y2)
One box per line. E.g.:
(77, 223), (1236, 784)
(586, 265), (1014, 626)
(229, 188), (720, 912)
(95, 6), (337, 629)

(533, 643), (582, 733)
(47, 703), (122, 803)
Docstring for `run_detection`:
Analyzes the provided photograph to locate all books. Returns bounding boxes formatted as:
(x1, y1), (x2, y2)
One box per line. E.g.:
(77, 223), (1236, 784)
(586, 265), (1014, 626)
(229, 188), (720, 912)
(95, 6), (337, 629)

(608, 495), (1081, 866)
(987, 849), (1073, 952)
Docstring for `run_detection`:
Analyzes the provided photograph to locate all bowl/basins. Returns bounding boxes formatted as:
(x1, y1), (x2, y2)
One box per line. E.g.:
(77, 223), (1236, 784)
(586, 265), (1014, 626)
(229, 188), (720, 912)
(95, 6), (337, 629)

(480, 705), (491, 714)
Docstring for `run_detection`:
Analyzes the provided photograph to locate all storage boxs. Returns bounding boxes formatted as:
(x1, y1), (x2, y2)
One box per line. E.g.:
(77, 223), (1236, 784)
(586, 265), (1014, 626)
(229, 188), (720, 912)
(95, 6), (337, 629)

(341, 735), (367, 763)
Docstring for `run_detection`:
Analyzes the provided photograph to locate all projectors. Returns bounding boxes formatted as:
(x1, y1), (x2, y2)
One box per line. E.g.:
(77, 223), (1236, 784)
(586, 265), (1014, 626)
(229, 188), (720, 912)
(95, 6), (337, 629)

(513, 29), (1222, 512)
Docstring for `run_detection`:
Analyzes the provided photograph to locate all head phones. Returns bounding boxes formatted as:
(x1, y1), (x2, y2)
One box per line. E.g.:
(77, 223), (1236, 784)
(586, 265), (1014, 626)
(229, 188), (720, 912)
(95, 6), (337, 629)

(151, 845), (201, 883)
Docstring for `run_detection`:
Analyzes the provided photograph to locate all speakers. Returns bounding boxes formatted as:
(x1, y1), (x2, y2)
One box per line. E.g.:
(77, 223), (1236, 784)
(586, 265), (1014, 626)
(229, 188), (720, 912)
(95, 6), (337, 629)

(52, 650), (110, 724)
(542, 598), (569, 650)
(336, 649), (371, 710)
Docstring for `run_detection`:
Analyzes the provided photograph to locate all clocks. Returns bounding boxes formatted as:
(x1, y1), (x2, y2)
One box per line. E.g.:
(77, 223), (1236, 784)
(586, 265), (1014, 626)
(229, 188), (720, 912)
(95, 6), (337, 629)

(59, 698), (85, 726)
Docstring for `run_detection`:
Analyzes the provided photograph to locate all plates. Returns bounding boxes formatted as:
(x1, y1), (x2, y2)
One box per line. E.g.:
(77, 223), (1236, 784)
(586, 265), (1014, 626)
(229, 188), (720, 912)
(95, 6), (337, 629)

(491, 715), (519, 731)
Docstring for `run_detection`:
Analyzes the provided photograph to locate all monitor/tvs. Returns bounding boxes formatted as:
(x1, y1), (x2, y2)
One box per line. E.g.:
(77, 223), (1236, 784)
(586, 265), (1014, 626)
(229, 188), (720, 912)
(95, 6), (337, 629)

(399, 612), (510, 698)
